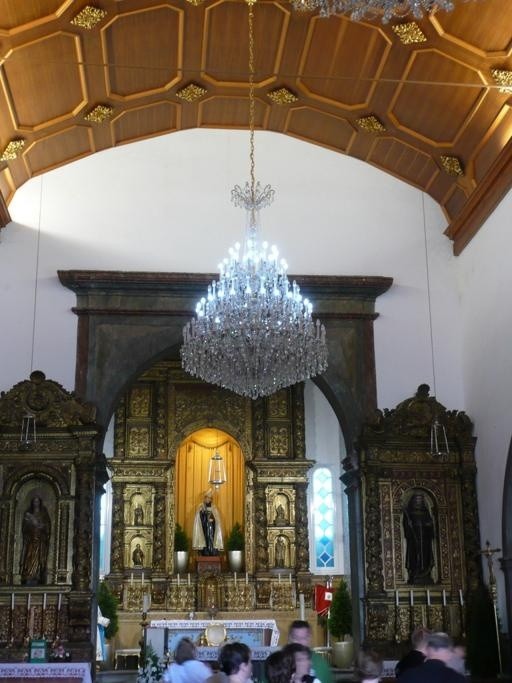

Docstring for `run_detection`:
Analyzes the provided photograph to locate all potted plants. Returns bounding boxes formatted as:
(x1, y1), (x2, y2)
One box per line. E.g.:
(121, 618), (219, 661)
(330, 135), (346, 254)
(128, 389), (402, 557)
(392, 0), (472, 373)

(174, 522), (191, 574)
(327, 578), (354, 670)
(95, 579), (120, 671)
(224, 520), (245, 573)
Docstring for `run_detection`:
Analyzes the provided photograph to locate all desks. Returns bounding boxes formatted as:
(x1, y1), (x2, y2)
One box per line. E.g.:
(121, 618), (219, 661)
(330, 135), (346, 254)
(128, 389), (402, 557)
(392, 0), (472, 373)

(194, 646), (282, 683)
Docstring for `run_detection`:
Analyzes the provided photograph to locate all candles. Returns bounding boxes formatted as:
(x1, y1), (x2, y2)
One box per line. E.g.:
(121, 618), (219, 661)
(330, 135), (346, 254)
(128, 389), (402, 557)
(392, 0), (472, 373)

(233, 571), (249, 587)
(10, 591), (63, 613)
(299, 593), (305, 621)
(395, 587), (465, 606)
(130, 571), (144, 588)
(143, 594), (147, 613)
(176, 571), (191, 586)
(278, 572), (292, 585)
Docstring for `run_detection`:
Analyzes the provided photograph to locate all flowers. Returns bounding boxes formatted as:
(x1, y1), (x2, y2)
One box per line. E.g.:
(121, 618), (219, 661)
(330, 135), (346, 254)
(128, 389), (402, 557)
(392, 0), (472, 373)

(136, 640), (168, 683)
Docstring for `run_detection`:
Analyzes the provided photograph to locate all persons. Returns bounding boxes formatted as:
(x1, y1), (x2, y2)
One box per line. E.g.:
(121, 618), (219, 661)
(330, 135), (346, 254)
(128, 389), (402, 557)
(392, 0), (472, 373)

(132, 504), (144, 526)
(395, 628), (430, 677)
(157, 635), (214, 682)
(275, 503), (284, 523)
(265, 650), (296, 683)
(289, 620), (311, 647)
(206, 642), (254, 683)
(395, 630), (467, 682)
(133, 544), (145, 568)
(20, 493), (51, 585)
(275, 535), (288, 569)
(446, 638), (466, 677)
(281, 643), (321, 683)
(353, 648), (384, 683)
(404, 491), (438, 585)
(198, 488), (219, 555)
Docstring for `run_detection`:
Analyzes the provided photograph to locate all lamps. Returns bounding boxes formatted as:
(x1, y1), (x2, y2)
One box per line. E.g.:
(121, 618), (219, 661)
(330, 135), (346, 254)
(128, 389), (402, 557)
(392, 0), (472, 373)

(177, 0), (331, 405)
(423, 180), (450, 456)
(207, 429), (227, 490)
(18, 118), (48, 453)
